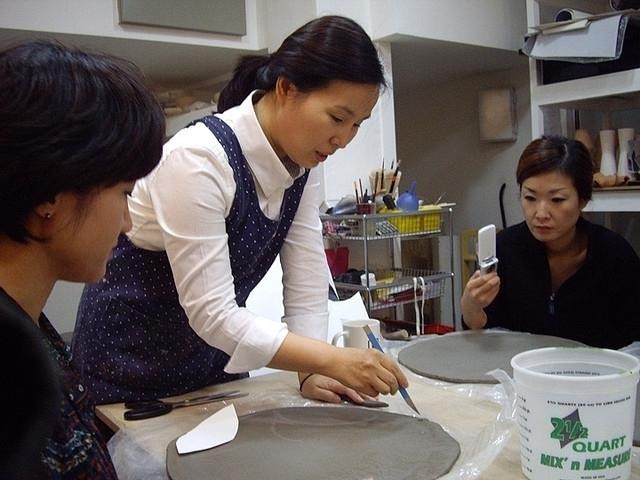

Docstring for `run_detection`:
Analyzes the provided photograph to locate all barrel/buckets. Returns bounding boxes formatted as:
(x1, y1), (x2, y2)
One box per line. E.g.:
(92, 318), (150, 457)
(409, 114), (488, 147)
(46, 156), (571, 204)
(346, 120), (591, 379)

(510, 346), (640, 480)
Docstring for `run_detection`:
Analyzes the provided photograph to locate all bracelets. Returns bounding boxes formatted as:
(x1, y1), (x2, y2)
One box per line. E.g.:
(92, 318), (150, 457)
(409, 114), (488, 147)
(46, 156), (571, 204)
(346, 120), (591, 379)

(298, 372), (315, 392)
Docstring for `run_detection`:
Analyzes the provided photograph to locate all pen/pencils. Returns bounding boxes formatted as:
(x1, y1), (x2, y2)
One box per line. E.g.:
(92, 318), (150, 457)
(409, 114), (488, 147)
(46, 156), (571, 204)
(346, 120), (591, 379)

(363, 325), (421, 416)
(354, 158), (402, 204)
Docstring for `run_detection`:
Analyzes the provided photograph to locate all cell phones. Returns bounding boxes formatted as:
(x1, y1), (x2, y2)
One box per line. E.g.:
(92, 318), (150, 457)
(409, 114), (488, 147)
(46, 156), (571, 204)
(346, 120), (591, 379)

(476, 224), (500, 277)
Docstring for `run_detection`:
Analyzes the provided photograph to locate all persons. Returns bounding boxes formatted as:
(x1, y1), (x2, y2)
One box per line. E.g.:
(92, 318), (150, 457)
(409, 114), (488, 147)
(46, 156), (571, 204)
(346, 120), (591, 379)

(1, 34), (168, 479)
(460, 133), (639, 350)
(70, 15), (410, 404)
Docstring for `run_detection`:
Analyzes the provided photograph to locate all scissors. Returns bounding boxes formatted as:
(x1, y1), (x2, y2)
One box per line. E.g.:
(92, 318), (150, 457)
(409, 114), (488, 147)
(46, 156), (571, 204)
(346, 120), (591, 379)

(125, 390), (249, 420)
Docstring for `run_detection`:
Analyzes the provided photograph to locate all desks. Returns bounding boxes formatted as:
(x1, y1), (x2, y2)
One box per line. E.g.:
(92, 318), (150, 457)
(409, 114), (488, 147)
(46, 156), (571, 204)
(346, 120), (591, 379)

(93, 348), (640, 480)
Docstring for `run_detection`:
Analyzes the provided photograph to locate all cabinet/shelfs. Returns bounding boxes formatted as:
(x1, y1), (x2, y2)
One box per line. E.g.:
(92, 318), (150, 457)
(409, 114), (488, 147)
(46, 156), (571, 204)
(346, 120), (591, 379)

(526, 0), (640, 214)
(319, 207), (457, 335)
